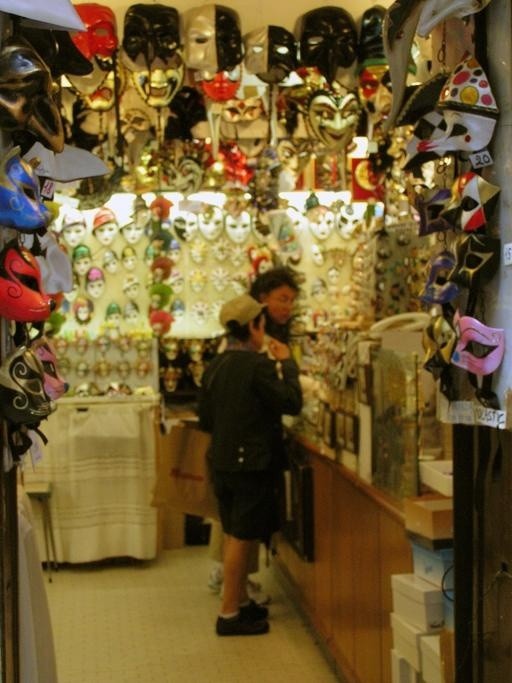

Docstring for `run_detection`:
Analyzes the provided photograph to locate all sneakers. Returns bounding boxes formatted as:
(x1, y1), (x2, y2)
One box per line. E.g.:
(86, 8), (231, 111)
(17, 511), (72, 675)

(216, 611), (269, 636)
(239, 598), (269, 618)
(214, 582), (273, 606)
(207, 563), (262, 591)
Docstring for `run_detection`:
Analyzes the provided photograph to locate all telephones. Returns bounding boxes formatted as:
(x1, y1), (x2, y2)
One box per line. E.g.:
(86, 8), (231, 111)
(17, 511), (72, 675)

(368, 312), (431, 340)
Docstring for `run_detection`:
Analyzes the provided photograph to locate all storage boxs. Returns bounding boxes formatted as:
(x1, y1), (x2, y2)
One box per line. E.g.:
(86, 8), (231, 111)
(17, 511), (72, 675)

(387, 496), (455, 683)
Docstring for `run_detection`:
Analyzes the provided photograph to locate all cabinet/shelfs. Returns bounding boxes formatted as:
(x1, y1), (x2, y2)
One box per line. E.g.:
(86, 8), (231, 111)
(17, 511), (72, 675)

(269, 420), (425, 682)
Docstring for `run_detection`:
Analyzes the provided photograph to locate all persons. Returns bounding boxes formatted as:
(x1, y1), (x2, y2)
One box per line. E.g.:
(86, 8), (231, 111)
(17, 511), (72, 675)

(198, 293), (303, 635)
(207, 268), (300, 604)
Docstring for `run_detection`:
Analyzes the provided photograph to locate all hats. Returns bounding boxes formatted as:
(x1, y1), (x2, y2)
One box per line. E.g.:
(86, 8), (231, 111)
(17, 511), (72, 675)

(218, 292), (268, 338)
